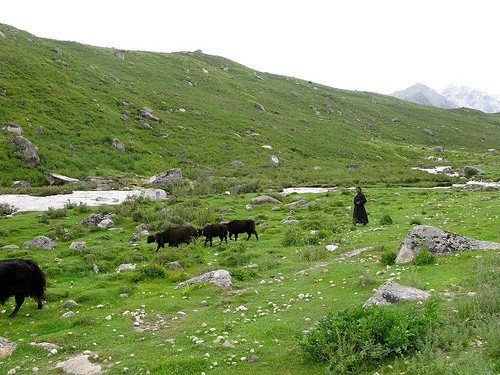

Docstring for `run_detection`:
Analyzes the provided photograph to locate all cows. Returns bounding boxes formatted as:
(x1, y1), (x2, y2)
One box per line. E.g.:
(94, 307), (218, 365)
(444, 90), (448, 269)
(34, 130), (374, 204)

(147, 227), (191, 252)
(222, 220), (258, 241)
(197, 225), (227, 247)
(167, 224), (197, 247)
(0, 259), (46, 319)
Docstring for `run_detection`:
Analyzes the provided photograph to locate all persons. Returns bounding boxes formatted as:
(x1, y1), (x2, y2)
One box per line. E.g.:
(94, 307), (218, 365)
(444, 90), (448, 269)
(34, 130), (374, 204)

(352, 187), (368, 227)
